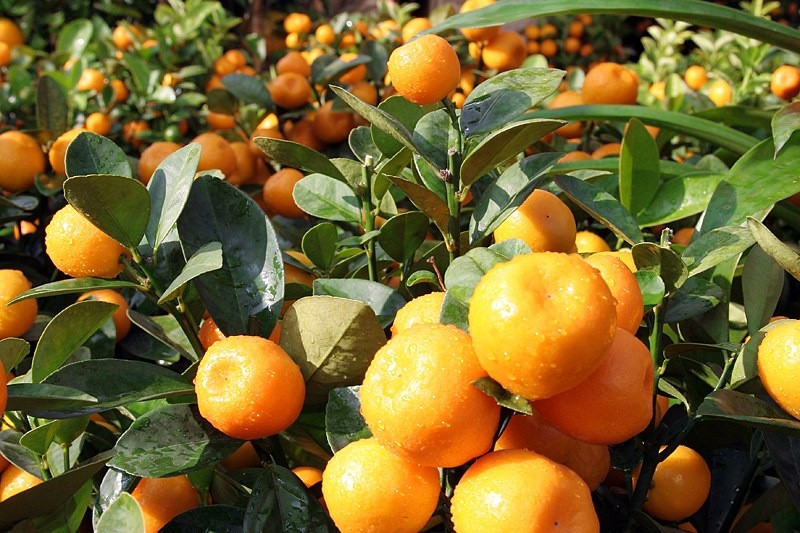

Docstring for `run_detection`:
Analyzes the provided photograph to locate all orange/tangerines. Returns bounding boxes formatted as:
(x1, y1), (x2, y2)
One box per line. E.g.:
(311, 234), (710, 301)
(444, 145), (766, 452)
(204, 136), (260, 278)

(0, 1), (800, 533)
